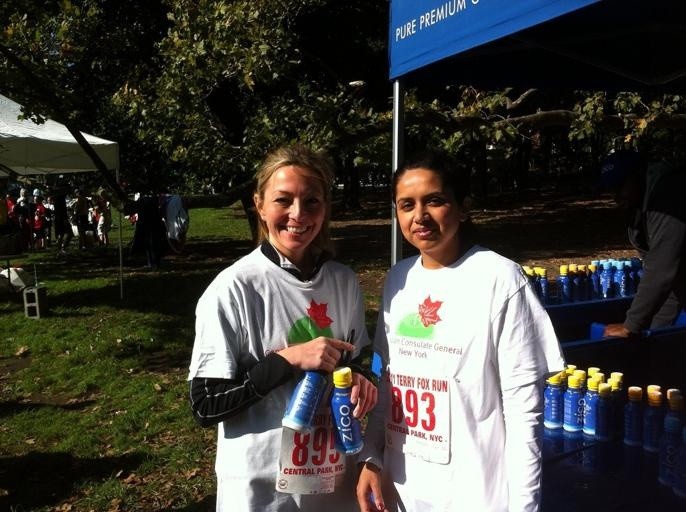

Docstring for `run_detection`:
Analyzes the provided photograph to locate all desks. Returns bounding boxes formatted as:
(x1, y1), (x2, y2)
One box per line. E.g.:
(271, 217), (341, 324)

(536, 293), (685, 512)
(0, 255), (28, 284)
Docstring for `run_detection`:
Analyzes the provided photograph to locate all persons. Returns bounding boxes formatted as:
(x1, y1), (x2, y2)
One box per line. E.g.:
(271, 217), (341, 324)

(0, 187), (111, 255)
(588, 151), (685, 339)
(355, 157), (575, 511)
(187, 144), (378, 511)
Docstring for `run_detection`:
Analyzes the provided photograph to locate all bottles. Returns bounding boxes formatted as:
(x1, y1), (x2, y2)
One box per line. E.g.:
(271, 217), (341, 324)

(523, 257), (685, 496)
(281, 367), (365, 458)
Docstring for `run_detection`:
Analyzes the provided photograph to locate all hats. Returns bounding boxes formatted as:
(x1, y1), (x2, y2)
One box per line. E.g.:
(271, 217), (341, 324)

(19, 188), (40, 197)
